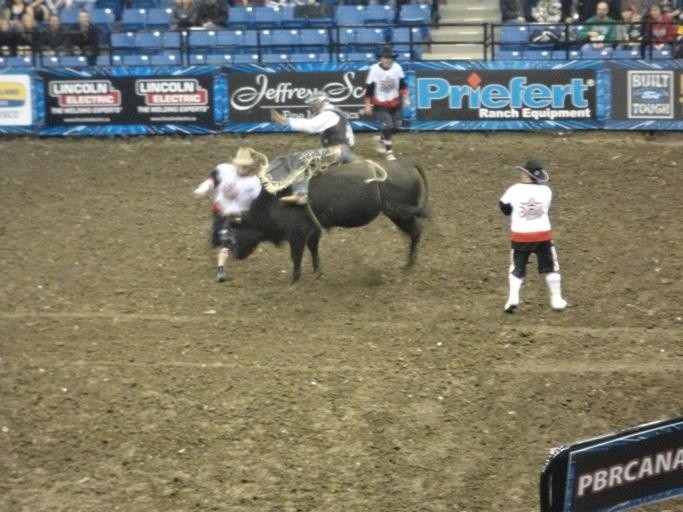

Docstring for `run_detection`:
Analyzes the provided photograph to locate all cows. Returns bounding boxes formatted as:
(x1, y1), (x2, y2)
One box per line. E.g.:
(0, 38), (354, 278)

(228, 158), (430, 286)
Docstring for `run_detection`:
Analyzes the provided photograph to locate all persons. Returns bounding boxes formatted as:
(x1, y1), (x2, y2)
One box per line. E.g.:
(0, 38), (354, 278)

(0, 0), (104, 66)
(499, 2), (683, 61)
(189, 143), (264, 283)
(363, 45), (411, 164)
(499, 160), (569, 315)
(266, 93), (358, 206)
(162, 0), (346, 32)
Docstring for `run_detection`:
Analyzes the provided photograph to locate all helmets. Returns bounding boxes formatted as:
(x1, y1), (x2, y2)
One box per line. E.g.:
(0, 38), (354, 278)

(304, 93), (326, 106)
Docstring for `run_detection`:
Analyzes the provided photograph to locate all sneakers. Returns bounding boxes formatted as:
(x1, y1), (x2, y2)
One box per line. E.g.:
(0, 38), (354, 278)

(551, 299), (568, 311)
(216, 266), (226, 282)
(373, 135), (398, 161)
(504, 302), (519, 312)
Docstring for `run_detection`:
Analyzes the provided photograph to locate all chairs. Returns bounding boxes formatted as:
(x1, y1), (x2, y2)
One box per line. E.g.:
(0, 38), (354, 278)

(0, 0), (436, 65)
(493, 23), (673, 61)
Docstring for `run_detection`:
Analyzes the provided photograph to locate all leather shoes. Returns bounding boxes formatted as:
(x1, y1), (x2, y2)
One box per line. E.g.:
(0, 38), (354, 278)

(279, 195), (308, 205)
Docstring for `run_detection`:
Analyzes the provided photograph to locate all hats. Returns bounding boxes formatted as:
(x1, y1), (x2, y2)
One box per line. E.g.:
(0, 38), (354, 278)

(376, 46), (399, 57)
(515, 159), (549, 183)
(232, 147), (256, 165)
